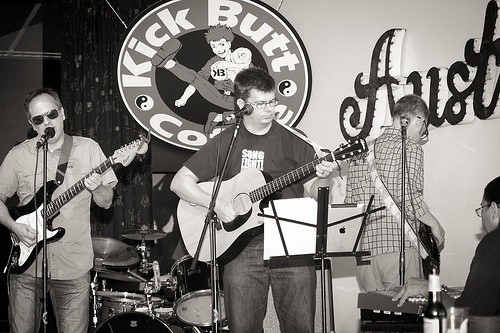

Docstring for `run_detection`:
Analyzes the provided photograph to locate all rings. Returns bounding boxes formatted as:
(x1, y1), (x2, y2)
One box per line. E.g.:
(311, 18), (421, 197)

(321, 170), (324, 174)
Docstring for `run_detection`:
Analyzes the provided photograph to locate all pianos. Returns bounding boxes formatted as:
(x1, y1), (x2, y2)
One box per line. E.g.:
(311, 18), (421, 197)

(357, 287), (466, 333)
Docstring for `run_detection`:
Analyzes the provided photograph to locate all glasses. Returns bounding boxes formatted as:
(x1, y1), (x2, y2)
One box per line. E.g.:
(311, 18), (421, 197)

(241, 96), (279, 109)
(475, 202), (500, 216)
(417, 116), (429, 139)
(30, 106), (62, 125)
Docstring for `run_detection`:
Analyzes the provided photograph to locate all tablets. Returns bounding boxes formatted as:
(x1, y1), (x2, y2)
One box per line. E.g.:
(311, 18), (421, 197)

(327, 203), (366, 253)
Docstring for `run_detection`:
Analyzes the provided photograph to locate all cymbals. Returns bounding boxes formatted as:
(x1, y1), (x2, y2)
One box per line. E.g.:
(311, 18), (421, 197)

(91, 236), (141, 266)
(119, 228), (166, 240)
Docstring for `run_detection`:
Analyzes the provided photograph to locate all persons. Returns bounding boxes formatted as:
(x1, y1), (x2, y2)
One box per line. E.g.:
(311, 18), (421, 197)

(0, 88), (118, 333)
(343, 94), (446, 322)
(169, 67), (342, 333)
(392, 176), (500, 333)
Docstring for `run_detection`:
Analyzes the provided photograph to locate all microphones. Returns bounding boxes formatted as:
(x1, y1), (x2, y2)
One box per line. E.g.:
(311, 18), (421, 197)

(224, 104), (254, 122)
(400, 113), (410, 130)
(37, 127), (56, 149)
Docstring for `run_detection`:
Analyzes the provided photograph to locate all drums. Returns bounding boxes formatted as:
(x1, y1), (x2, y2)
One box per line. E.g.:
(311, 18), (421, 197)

(170, 253), (227, 327)
(90, 269), (140, 293)
(93, 310), (201, 333)
(87, 291), (164, 333)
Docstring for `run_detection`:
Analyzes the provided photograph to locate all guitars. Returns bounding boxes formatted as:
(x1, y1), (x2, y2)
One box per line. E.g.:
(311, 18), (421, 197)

(177, 134), (369, 266)
(7, 139), (148, 274)
(418, 224), (441, 279)
